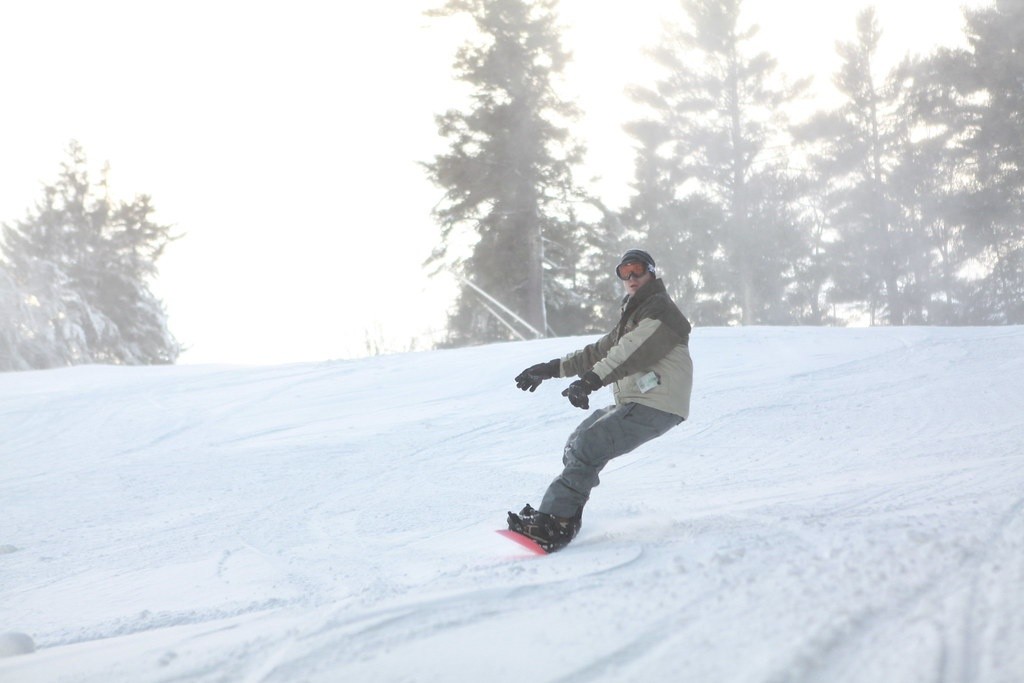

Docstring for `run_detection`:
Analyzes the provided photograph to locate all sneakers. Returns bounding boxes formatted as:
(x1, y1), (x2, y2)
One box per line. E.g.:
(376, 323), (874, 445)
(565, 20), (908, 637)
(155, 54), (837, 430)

(506, 513), (574, 545)
(519, 502), (582, 540)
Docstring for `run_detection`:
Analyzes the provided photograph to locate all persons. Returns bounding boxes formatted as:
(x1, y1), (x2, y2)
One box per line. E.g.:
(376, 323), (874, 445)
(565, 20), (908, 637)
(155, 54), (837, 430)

(506, 250), (693, 553)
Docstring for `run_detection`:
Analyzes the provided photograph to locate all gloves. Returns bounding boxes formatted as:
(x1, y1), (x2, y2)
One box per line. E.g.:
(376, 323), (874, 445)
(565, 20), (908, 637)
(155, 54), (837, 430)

(515, 359), (561, 393)
(561, 370), (602, 409)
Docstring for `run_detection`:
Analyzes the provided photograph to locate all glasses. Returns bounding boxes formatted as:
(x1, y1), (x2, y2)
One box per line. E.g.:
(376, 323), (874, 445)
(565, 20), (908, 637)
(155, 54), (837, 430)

(616, 262), (648, 281)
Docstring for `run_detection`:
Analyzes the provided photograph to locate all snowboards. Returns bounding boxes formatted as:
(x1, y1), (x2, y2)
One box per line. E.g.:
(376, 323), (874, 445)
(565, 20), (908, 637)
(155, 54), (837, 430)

(495, 526), (551, 556)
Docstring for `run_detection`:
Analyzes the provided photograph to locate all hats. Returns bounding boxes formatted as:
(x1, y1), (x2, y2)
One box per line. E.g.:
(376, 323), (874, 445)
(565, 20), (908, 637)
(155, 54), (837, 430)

(620, 248), (656, 272)
